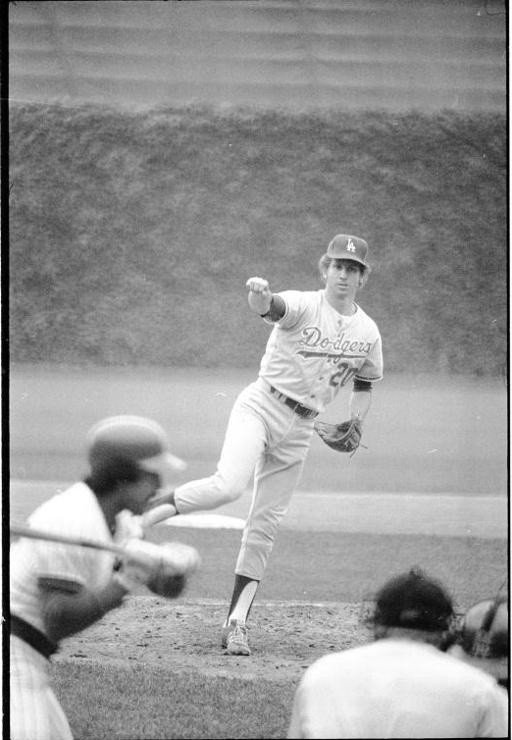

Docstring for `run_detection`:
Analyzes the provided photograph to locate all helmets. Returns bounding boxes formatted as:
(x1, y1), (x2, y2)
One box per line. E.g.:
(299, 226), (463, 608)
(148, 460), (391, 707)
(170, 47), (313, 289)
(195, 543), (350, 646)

(88, 415), (188, 476)
(445, 599), (508, 679)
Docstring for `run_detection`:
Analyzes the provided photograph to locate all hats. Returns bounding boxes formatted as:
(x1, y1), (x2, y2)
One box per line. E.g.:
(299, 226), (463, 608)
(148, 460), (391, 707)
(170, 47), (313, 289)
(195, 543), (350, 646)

(364, 569), (457, 632)
(327, 234), (369, 270)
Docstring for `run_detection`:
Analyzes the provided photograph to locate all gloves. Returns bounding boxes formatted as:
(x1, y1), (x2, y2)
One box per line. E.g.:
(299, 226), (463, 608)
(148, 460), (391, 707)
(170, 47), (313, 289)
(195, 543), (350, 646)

(114, 538), (199, 595)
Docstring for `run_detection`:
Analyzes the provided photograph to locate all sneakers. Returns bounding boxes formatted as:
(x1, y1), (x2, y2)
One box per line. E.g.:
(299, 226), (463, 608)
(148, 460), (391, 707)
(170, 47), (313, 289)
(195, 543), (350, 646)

(222, 619), (251, 656)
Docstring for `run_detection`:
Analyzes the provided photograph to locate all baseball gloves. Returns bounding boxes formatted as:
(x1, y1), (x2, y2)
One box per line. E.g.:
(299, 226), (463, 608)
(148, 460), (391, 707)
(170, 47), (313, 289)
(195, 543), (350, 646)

(315, 418), (363, 452)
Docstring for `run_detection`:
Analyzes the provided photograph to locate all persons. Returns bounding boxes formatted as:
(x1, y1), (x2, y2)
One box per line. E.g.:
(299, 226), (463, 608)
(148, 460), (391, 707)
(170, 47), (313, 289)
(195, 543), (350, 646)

(140, 234), (382, 656)
(441, 599), (509, 688)
(9, 414), (185, 739)
(288, 574), (510, 740)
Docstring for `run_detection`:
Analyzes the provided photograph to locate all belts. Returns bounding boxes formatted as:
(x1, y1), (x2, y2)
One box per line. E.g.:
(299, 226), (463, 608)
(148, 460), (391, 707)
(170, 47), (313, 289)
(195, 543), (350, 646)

(270, 387), (319, 418)
